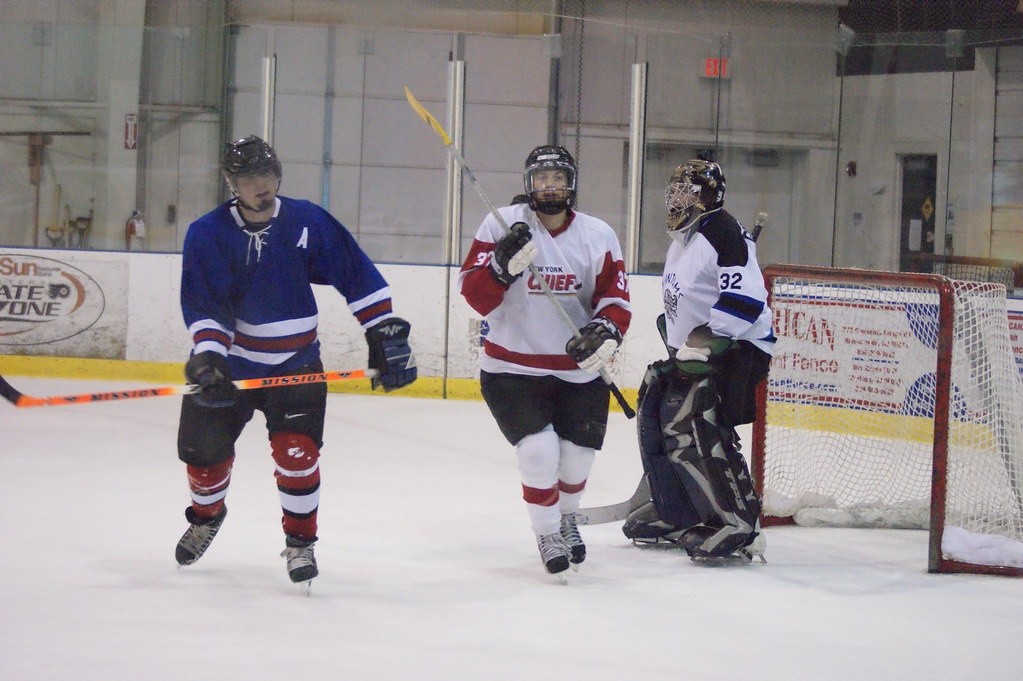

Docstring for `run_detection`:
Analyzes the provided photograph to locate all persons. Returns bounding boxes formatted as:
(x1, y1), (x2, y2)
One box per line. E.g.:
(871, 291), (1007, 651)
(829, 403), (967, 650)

(175, 135), (418, 598)
(455, 144), (633, 585)
(623, 159), (777, 564)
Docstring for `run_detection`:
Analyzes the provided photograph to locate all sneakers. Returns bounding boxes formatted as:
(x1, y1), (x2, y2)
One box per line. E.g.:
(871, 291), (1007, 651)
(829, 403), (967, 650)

(536, 529), (569, 585)
(175, 506), (227, 566)
(558, 513), (588, 573)
(280, 534), (319, 595)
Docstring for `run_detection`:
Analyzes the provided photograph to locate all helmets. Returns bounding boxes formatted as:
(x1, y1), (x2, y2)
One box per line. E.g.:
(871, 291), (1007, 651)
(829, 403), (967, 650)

(222, 134), (282, 192)
(522, 145), (576, 215)
(667, 159), (725, 231)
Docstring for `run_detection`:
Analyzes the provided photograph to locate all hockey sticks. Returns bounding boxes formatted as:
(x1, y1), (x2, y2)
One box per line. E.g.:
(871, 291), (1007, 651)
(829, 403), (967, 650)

(576, 212), (766, 527)
(0, 369), (381, 408)
(402, 85), (635, 419)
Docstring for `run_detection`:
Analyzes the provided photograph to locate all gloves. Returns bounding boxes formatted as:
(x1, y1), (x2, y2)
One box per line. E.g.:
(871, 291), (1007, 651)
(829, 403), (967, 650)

(365, 318), (417, 392)
(185, 350), (237, 408)
(566, 316), (622, 375)
(488, 223), (537, 286)
